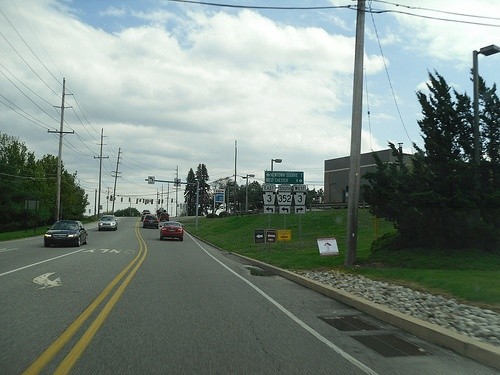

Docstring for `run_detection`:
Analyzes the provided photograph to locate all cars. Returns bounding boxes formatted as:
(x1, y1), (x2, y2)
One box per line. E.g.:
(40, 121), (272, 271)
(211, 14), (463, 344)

(159, 212), (170, 222)
(141, 209), (160, 229)
(44, 220), (89, 247)
(160, 221), (184, 242)
(98, 214), (119, 231)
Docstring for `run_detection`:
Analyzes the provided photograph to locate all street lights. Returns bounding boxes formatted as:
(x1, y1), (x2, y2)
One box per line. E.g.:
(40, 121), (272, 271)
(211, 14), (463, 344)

(472, 43), (500, 166)
(270, 158), (283, 171)
(245, 174), (256, 214)
